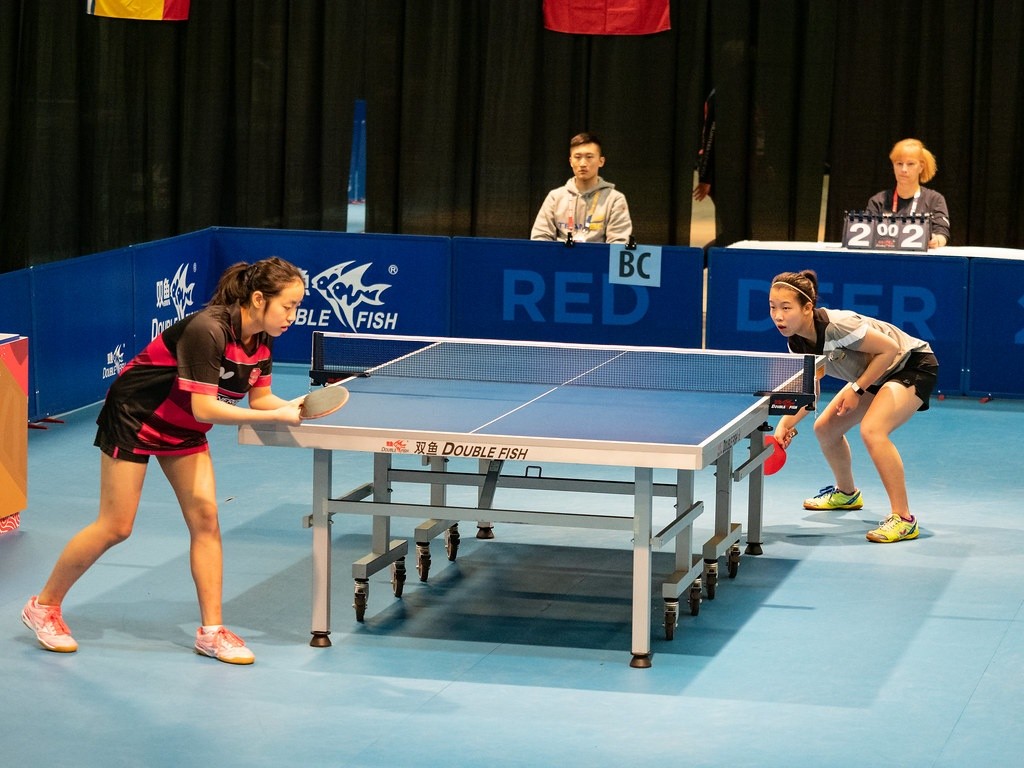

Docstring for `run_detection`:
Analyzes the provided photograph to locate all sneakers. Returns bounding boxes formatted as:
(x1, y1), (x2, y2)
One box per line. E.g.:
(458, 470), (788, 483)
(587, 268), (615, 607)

(21, 595), (78, 652)
(866, 514), (919, 543)
(194, 627), (255, 664)
(803, 485), (862, 510)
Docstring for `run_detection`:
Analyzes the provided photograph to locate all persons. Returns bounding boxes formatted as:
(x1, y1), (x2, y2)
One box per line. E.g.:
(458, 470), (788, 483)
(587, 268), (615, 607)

(768, 268), (940, 542)
(692, 30), (759, 269)
(865, 138), (952, 249)
(20, 256), (311, 664)
(529, 132), (633, 244)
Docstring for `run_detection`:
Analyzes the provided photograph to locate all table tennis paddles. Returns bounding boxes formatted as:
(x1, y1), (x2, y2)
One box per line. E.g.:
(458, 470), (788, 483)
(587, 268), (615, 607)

(297, 384), (351, 421)
(761, 426), (799, 477)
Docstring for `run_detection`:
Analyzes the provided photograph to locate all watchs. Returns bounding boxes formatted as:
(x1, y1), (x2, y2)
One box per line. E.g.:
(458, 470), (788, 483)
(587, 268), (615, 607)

(851, 382), (866, 397)
